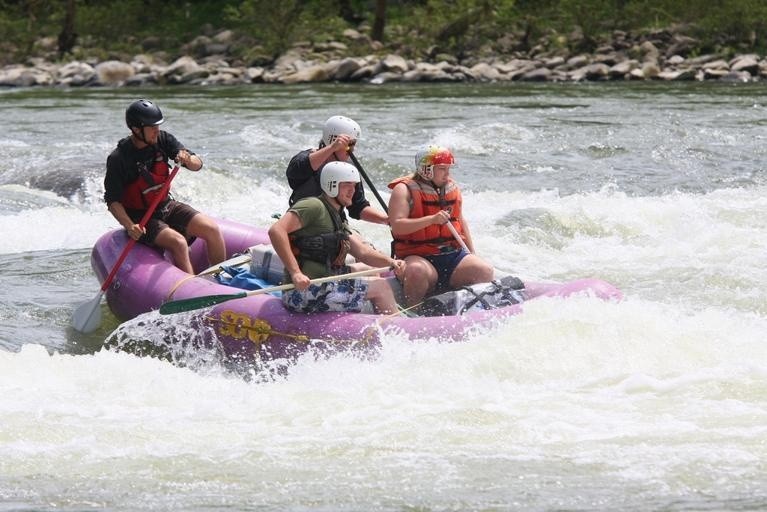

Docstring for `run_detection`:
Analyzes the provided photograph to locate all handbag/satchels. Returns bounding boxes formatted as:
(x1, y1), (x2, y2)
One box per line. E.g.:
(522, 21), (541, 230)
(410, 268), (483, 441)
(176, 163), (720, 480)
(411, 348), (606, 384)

(421, 275), (525, 317)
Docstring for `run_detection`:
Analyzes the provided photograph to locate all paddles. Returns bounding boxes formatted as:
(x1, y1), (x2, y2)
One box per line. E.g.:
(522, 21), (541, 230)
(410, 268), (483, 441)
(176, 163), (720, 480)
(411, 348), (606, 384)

(159, 266), (395, 315)
(72, 161), (182, 334)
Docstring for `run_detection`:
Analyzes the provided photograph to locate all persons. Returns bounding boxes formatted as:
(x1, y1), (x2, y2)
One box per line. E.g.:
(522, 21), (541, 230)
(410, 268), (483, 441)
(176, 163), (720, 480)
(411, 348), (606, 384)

(103, 98), (226, 275)
(285, 114), (390, 226)
(386, 142), (494, 306)
(266, 161), (406, 315)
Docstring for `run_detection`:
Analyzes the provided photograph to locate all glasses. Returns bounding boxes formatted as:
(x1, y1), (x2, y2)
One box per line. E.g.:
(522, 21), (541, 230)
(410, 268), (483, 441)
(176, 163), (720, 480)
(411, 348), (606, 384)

(348, 141), (356, 146)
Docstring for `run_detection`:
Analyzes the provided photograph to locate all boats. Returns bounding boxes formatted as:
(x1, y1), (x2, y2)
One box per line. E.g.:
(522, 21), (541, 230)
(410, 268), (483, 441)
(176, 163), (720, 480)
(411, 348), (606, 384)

(87, 213), (629, 388)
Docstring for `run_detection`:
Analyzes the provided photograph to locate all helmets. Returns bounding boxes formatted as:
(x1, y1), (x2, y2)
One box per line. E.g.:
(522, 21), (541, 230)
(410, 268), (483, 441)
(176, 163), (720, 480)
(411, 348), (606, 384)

(320, 160), (361, 198)
(126, 99), (165, 126)
(416, 143), (455, 181)
(322, 115), (362, 147)
